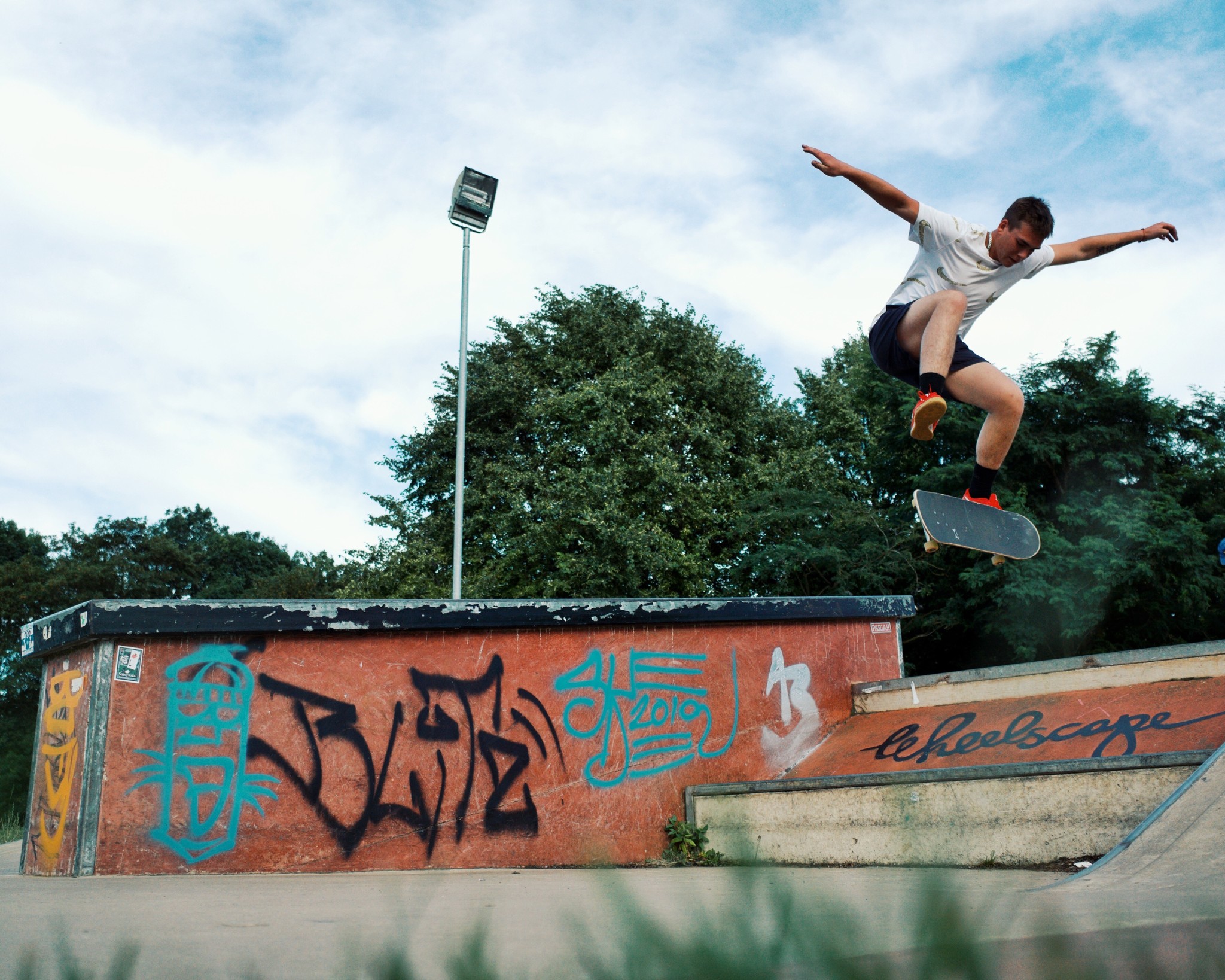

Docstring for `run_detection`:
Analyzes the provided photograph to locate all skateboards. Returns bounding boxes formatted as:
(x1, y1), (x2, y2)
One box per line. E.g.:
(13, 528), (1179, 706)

(911, 489), (1040, 566)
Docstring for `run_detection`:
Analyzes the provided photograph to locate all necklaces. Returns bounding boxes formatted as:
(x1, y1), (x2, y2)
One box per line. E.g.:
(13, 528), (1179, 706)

(987, 231), (992, 251)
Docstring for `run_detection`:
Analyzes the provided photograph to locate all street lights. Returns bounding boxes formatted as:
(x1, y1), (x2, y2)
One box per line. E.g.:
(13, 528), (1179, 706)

(446, 167), (500, 601)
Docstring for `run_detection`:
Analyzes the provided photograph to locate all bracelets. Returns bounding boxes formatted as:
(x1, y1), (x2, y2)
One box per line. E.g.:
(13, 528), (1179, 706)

(1138, 228), (1144, 243)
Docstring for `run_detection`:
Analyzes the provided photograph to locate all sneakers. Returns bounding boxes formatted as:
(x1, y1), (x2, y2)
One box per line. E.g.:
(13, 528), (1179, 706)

(962, 487), (1002, 510)
(910, 383), (947, 441)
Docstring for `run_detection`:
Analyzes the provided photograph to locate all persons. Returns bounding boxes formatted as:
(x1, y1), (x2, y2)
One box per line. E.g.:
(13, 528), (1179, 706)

(801, 144), (1179, 511)
